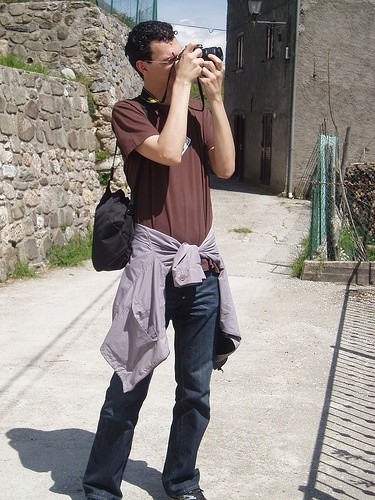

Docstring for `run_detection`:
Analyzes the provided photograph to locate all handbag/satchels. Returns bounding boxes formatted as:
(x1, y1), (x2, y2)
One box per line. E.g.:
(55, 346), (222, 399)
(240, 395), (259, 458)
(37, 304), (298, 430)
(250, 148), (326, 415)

(91, 185), (134, 271)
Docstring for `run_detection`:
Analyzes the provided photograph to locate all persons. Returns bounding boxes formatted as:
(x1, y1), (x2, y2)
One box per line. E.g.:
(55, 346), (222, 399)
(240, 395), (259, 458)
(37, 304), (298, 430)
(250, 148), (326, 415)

(81, 20), (235, 499)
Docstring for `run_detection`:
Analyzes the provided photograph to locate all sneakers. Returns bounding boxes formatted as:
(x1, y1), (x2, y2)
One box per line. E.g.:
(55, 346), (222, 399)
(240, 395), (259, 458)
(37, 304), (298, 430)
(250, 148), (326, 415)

(164, 486), (207, 500)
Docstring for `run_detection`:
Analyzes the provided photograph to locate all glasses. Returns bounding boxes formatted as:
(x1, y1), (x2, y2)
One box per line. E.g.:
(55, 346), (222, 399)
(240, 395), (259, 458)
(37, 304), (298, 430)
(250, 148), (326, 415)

(145, 55), (180, 65)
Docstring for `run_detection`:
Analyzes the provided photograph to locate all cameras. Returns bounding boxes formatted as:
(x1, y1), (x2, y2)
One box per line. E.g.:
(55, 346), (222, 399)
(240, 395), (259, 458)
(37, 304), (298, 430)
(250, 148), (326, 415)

(192, 44), (224, 71)
